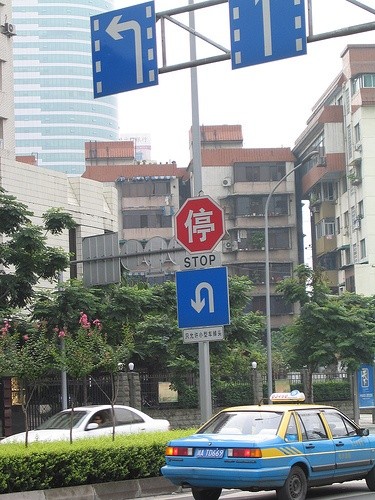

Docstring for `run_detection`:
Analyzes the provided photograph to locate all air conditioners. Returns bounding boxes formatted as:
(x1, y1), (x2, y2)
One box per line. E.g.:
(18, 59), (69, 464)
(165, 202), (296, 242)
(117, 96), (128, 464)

(222, 177), (233, 187)
(224, 241), (232, 249)
(316, 156), (326, 167)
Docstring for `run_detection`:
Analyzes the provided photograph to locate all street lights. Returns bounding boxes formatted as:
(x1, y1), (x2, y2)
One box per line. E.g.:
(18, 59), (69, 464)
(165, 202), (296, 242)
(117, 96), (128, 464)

(263, 150), (321, 403)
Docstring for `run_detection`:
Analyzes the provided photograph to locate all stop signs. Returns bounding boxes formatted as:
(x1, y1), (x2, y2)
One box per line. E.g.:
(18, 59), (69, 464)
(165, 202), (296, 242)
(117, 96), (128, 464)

(174, 195), (225, 254)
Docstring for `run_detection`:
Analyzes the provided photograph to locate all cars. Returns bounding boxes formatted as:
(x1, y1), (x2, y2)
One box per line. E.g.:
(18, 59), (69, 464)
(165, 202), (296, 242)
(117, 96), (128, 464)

(161, 390), (375, 500)
(1, 404), (171, 441)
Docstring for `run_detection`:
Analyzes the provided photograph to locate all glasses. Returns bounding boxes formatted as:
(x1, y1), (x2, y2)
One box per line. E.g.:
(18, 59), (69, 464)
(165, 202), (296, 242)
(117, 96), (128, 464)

(93, 418), (101, 421)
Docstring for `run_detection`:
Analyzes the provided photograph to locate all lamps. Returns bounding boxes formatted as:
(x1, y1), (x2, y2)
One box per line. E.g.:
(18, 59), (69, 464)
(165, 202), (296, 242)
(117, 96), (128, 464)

(117, 362), (124, 371)
(128, 362), (135, 372)
(251, 361), (257, 371)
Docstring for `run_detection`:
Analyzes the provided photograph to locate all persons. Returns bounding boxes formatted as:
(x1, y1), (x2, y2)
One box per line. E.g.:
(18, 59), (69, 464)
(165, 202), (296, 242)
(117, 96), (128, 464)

(93, 413), (103, 424)
